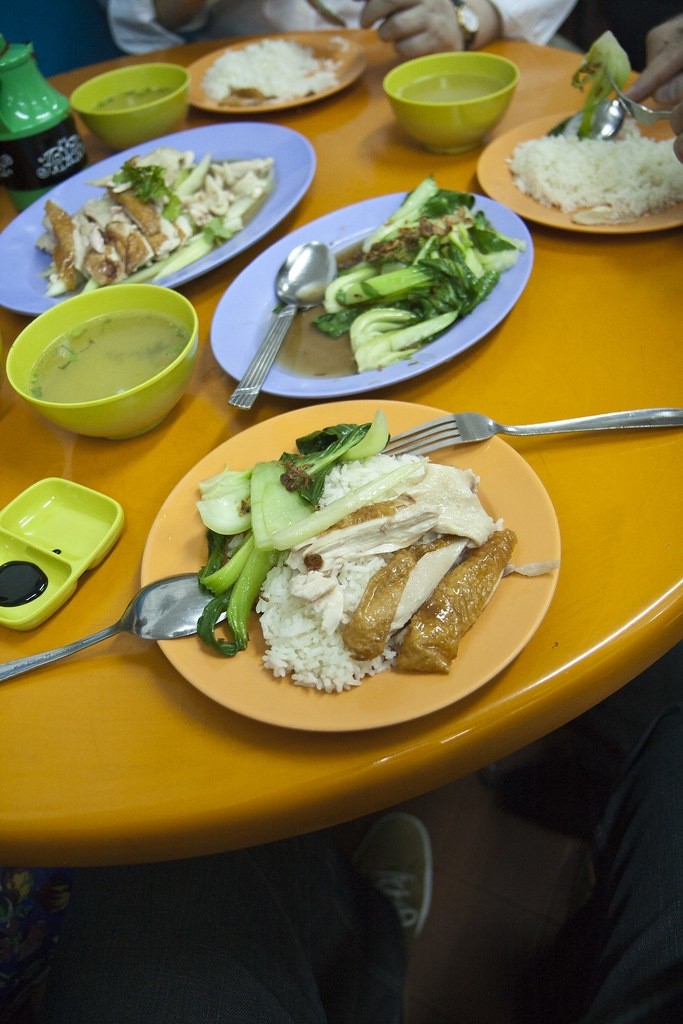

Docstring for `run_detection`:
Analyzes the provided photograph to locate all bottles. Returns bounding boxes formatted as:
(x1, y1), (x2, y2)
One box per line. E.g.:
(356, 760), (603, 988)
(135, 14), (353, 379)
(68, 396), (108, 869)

(0, 33), (90, 212)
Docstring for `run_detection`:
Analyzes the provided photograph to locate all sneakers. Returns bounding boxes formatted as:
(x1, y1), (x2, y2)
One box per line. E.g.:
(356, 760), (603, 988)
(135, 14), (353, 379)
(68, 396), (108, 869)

(353, 813), (434, 940)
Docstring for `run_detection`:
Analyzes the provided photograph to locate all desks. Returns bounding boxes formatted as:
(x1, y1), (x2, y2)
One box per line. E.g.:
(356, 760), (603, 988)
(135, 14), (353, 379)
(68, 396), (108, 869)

(0, 29), (683, 874)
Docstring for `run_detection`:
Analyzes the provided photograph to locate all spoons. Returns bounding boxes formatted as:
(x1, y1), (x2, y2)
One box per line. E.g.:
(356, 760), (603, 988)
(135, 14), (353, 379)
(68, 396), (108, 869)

(577, 93), (630, 141)
(0, 571), (230, 684)
(228, 242), (336, 413)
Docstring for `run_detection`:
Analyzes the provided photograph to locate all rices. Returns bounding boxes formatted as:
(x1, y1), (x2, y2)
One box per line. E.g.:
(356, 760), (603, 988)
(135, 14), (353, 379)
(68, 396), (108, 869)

(255, 452), (501, 693)
(506, 116), (683, 220)
(201, 34), (350, 105)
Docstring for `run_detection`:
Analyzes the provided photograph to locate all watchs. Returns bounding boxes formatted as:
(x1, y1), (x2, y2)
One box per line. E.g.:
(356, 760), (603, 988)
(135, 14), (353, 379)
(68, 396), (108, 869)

(451, 0), (479, 51)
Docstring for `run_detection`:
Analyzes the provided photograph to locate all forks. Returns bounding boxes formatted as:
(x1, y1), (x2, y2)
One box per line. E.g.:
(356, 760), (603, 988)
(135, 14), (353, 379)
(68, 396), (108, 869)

(381, 406), (682, 457)
(604, 67), (672, 125)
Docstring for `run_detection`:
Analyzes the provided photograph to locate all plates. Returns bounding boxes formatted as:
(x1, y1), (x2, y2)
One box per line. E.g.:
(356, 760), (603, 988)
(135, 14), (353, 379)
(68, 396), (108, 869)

(186, 32), (368, 114)
(142, 399), (563, 734)
(0, 121), (317, 317)
(475, 105), (683, 235)
(210, 187), (535, 399)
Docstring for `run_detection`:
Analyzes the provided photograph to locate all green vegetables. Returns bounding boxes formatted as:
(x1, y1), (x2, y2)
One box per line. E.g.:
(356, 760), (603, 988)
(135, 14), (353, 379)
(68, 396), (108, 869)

(81, 161), (237, 293)
(270, 172), (526, 373)
(193, 409), (428, 655)
(546, 29), (630, 141)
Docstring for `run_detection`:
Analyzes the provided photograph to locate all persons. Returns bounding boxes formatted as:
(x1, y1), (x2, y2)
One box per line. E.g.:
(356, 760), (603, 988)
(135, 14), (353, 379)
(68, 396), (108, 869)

(0, 702), (683, 1023)
(109, 0), (579, 61)
(557, 0), (683, 162)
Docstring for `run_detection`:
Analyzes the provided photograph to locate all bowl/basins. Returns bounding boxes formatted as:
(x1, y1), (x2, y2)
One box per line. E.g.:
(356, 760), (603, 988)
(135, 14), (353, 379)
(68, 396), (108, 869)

(69, 63), (191, 155)
(6, 283), (198, 441)
(383, 51), (520, 154)
